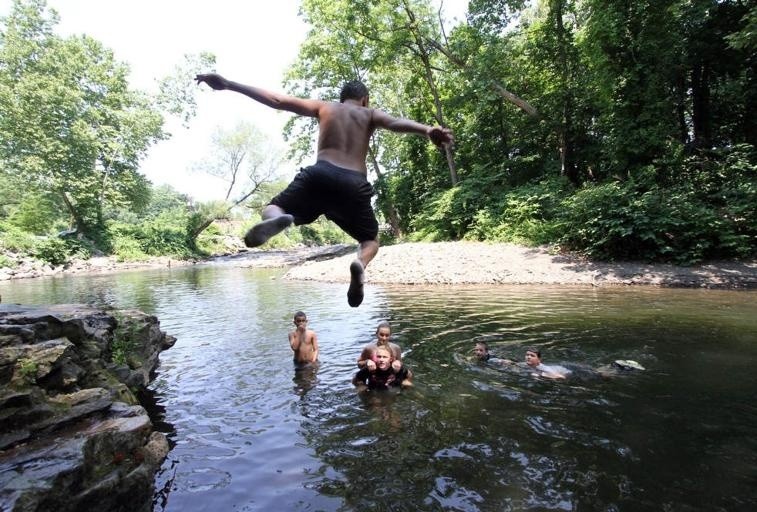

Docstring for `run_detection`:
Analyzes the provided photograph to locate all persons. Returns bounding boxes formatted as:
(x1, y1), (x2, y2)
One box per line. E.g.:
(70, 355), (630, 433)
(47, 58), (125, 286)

(503, 346), (568, 381)
(460, 341), (511, 369)
(193, 74), (454, 308)
(288, 310), (319, 365)
(356, 321), (404, 372)
(352, 345), (413, 395)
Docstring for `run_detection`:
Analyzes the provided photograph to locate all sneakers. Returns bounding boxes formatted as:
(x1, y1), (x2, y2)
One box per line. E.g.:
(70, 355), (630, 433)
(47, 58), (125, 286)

(614, 359), (646, 371)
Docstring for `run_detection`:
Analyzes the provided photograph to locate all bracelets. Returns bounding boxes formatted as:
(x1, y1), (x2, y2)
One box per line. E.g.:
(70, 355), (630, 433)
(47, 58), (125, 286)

(365, 359), (370, 366)
